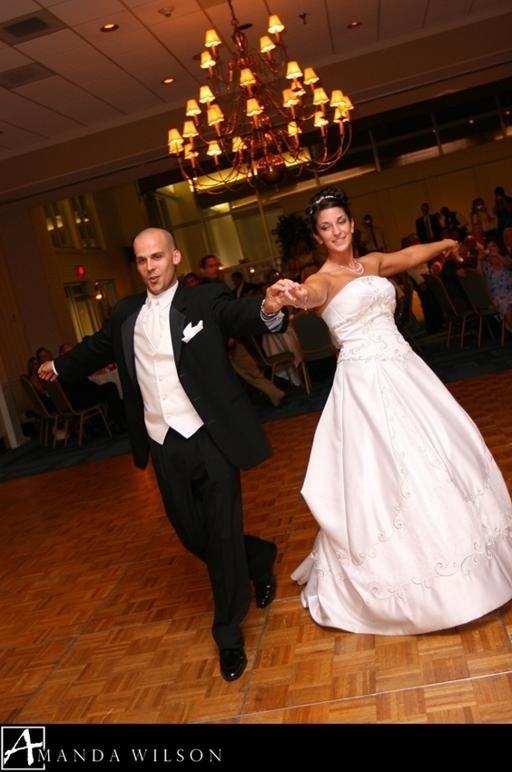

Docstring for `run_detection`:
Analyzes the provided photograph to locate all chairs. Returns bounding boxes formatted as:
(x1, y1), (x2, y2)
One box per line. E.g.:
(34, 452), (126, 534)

(13, 269), (512, 449)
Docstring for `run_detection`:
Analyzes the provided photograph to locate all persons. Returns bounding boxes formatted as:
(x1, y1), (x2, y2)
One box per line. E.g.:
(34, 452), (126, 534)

(271, 183), (512, 634)
(25, 185), (512, 432)
(38, 226), (302, 684)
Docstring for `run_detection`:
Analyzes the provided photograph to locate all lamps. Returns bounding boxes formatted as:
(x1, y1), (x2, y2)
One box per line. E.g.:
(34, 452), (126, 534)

(44, 195), (90, 232)
(167, 0), (355, 195)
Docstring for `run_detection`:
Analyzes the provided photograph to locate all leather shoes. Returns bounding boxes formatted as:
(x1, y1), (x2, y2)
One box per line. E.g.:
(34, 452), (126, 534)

(256, 578), (276, 607)
(220, 648), (247, 681)
